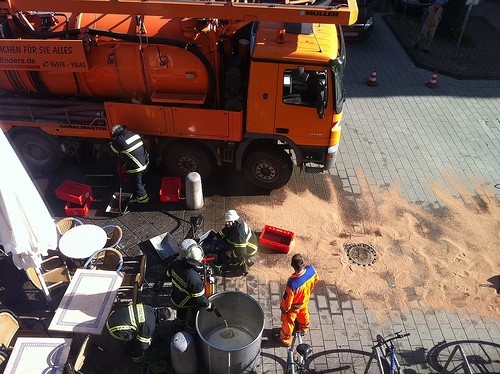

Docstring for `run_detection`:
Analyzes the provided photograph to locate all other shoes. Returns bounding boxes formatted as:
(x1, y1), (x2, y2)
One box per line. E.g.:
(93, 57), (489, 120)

(272, 332), (290, 347)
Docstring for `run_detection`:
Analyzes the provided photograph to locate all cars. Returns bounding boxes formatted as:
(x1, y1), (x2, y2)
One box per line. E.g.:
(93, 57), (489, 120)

(342, 0), (375, 38)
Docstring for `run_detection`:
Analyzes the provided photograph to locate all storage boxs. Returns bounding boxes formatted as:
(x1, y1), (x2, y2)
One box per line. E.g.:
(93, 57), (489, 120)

(259, 226), (296, 253)
(159, 176), (183, 202)
(55, 179), (91, 205)
(64, 193), (95, 217)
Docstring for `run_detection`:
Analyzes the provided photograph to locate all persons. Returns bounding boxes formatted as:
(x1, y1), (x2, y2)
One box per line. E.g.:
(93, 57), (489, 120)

(280, 253), (319, 346)
(415, 2), (443, 53)
(106, 303), (175, 364)
(213, 210), (259, 274)
(166, 245), (215, 335)
(109, 125), (149, 203)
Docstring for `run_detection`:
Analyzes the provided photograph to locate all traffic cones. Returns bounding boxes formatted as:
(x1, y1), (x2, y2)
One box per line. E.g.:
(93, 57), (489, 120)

(367, 69), (377, 86)
(427, 70), (439, 88)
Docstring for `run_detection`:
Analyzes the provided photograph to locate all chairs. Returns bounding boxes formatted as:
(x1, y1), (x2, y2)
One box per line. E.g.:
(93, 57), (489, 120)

(0, 214), (147, 374)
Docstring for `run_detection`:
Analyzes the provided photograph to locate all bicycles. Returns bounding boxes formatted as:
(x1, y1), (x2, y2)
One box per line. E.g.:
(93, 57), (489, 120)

(372, 329), (411, 374)
(285, 317), (313, 374)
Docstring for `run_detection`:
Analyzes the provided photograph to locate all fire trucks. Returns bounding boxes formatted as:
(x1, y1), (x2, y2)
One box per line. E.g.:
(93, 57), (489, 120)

(0, 0), (359, 191)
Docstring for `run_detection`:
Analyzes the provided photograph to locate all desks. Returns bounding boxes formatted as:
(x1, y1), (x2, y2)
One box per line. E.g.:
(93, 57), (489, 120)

(60, 225), (107, 260)
(2, 336), (75, 374)
(46, 268), (126, 335)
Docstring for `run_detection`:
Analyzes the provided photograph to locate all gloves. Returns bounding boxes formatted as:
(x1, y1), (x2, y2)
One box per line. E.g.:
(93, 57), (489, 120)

(205, 302), (215, 312)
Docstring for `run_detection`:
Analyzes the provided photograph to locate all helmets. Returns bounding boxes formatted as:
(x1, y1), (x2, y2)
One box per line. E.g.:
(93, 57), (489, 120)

(178, 237), (197, 258)
(186, 244), (205, 265)
(111, 124), (123, 136)
(225, 209), (239, 223)
